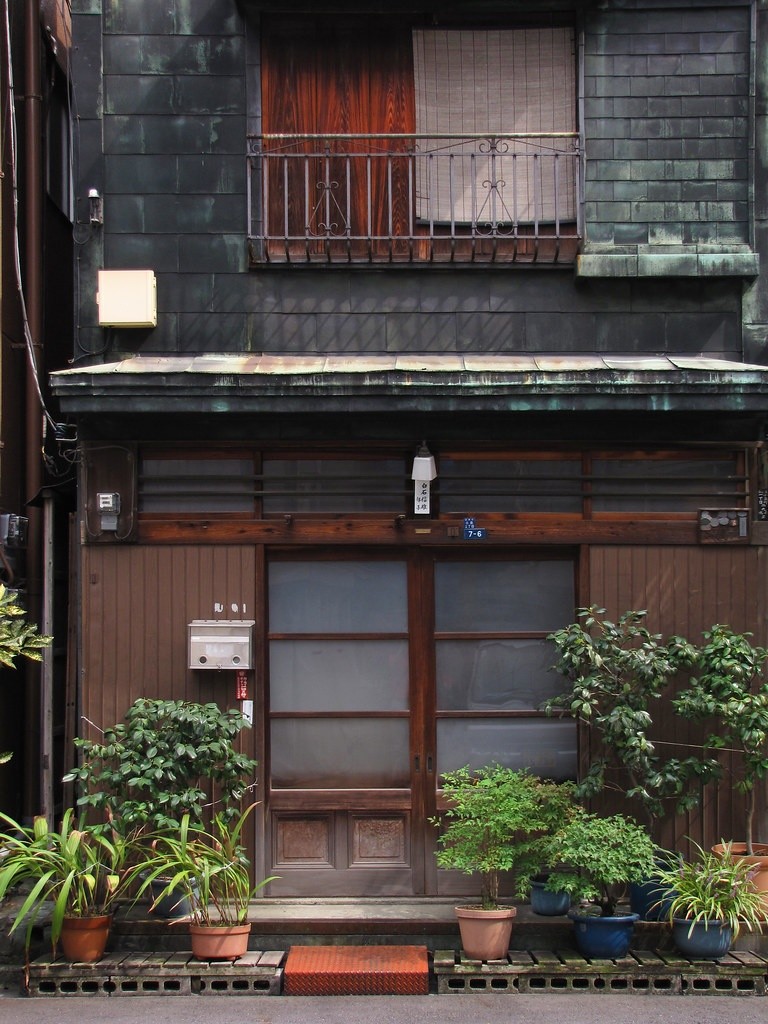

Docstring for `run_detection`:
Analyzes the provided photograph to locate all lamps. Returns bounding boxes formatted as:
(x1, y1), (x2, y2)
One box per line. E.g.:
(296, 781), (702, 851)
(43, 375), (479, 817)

(411, 440), (437, 481)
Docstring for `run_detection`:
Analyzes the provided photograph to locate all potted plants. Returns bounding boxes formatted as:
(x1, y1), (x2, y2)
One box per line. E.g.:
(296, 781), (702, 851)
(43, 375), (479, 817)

(427, 604), (768, 960)
(0, 698), (282, 995)
